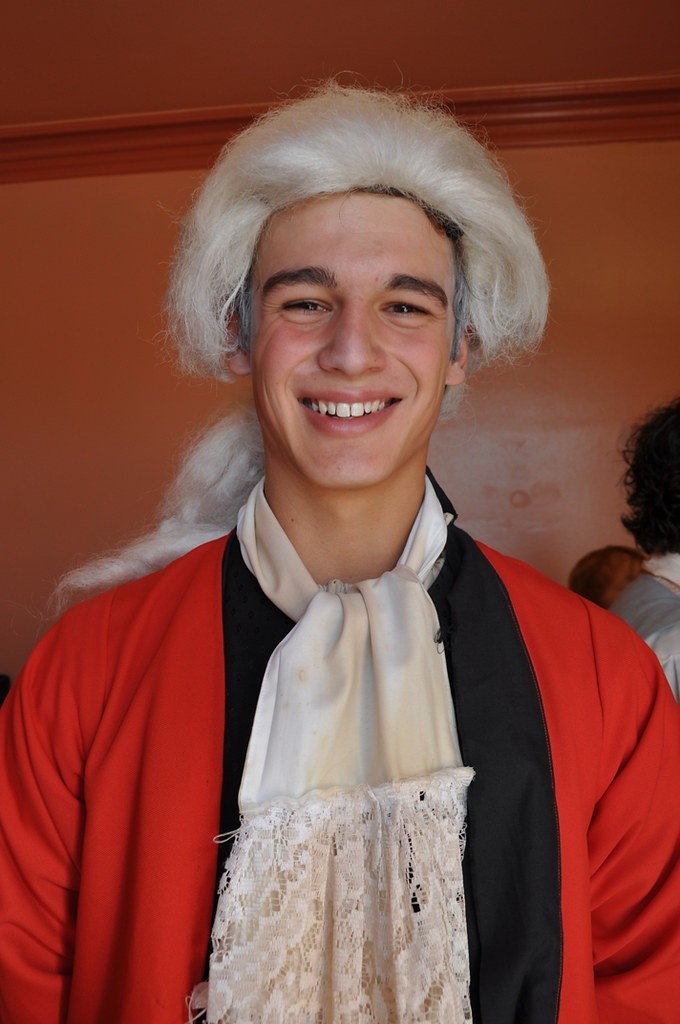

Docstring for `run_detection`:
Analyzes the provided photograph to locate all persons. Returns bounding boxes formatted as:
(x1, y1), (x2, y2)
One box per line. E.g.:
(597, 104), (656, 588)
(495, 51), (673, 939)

(568, 545), (646, 611)
(607, 397), (680, 707)
(0, 92), (680, 1024)
